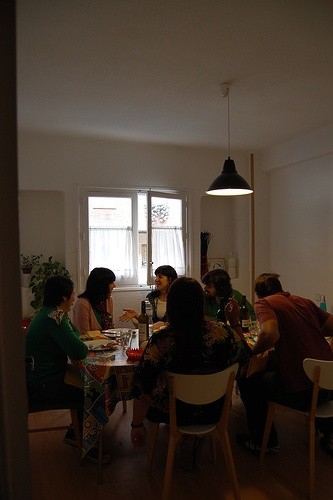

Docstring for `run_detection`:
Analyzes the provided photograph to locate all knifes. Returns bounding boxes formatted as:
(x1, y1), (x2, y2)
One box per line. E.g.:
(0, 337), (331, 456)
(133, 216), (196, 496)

(102, 331), (117, 334)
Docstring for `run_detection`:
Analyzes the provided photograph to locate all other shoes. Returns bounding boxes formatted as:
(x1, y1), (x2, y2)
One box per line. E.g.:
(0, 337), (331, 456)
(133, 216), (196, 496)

(243, 436), (287, 456)
(63, 425), (112, 464)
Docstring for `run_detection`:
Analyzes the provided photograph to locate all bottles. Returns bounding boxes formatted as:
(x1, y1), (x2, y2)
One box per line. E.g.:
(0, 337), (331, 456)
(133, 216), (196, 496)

(136, 300), (149, 347)
(238, 295), (250, 334)
(319, 296), (327, 312)
(216, 297), (227, 325)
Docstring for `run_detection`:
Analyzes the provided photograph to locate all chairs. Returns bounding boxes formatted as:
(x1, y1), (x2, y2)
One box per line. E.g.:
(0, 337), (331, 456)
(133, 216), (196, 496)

(258, 357), (333, 500)
(142, 363), (241, 500)
(29, 403), (82, 456)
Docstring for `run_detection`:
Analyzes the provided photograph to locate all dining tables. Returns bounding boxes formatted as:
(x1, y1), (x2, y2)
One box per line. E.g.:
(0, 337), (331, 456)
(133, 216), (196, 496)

(78, 326), (269, 414)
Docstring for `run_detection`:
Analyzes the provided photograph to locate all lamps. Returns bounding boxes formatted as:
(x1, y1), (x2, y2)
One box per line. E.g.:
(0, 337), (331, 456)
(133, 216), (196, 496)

(202, 82), (255, 196)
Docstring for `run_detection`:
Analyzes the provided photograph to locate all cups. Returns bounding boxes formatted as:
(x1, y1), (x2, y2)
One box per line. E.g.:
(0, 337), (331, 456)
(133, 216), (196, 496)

(118, 328), (133, 354)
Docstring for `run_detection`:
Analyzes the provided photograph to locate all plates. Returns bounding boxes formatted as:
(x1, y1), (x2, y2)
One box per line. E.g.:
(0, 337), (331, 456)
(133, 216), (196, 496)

(82, 340), (117, 351)
(104, 328), (134, 338)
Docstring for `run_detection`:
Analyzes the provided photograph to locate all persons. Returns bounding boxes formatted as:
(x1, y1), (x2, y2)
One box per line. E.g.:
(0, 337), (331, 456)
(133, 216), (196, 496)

(23, 276), (90, 447)
(201, 268), (259, 328)
(118, 264), (179, 325)
(72, 266), (118, 337)
(236, 272), (333, 455)
(130, 277), (254, 451)
